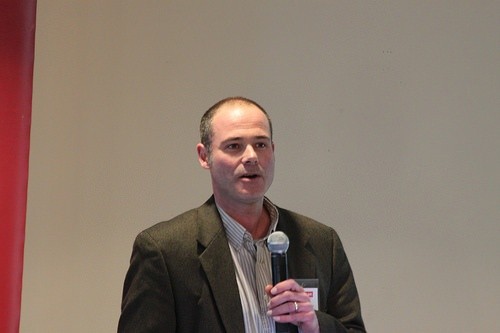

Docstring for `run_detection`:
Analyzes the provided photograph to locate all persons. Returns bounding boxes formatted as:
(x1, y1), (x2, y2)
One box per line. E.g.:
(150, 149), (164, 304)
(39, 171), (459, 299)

(117, 96), (367, 333)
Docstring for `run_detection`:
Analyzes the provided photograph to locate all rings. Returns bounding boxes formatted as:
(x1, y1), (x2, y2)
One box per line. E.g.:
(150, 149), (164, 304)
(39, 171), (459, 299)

(295, 301), (298, 311)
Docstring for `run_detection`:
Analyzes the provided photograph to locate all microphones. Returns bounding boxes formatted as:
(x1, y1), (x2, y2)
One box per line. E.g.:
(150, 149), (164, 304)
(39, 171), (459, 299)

(267, 231), (289, 333)
